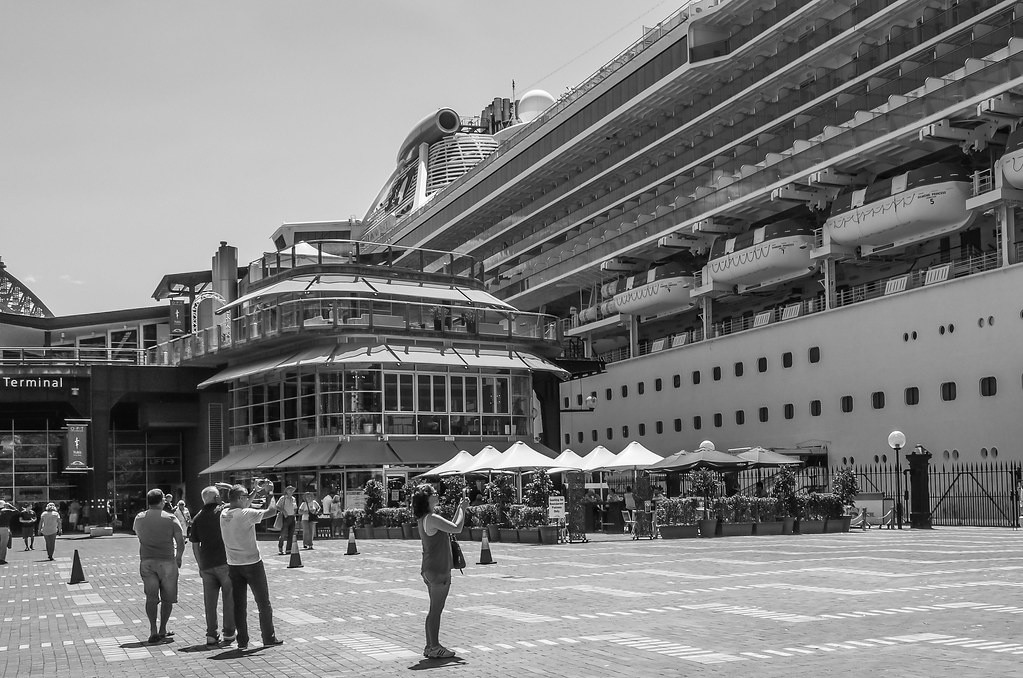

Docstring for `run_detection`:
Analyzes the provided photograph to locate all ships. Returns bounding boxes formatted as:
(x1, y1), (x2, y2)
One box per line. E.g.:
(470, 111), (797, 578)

(251, 0), (1023, 533)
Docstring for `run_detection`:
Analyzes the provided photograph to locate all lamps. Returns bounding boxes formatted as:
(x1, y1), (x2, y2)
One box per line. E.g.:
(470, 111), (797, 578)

(560, 395), (598, 412)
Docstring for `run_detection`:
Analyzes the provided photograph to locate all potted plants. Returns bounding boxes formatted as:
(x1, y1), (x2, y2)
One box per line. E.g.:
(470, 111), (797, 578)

(464, 311), (481, 332)
(342, 466), (860, 560)
(428, 306), (449, 330)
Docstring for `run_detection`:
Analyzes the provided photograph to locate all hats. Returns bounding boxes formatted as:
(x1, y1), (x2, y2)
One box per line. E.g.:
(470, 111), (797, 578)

(284, 486), (297, 496)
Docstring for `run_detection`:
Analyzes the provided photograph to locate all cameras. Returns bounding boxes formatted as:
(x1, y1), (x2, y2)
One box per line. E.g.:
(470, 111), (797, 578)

(258, 480), (269, 487)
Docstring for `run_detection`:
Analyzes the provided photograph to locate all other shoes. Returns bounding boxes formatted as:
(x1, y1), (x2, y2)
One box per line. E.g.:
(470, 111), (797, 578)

(149, 630), (175, 643)
(224, 630), (237, 642)
(207, 633), (223, 646)
(238, 643), (248, 650)
(264, 639), (283, 646)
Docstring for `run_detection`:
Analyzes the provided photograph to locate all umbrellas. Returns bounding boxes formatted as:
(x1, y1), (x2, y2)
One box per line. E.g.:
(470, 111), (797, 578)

(414, 440), (804, 504)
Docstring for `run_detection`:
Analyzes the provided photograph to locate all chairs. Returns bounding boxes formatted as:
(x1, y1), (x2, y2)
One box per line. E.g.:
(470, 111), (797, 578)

(632, 510), (644, 535)
(620, 510), (637, 535)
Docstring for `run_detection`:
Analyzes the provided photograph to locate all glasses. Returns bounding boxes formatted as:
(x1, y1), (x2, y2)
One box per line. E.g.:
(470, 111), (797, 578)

(240, 494), (250, 499)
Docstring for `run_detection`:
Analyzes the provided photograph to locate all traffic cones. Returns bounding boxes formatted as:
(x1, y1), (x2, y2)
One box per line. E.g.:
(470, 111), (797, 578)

(475, 529), (498, 564)
(344, 527), (360, 555)
(287, 534), (304, 568)
(67, 550), (89, 585)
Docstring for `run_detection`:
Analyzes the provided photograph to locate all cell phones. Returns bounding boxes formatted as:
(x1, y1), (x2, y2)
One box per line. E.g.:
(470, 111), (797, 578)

(462, 488), (467, 500)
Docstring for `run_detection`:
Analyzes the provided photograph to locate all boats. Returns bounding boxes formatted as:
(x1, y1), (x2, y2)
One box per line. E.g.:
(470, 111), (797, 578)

(704, 215), (824, 297)
(601, 258), (703, 327)
(826, 159), (982, 258)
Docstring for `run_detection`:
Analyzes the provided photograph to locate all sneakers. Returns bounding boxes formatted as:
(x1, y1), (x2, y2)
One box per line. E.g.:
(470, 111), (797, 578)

(426, 645), (456, 658)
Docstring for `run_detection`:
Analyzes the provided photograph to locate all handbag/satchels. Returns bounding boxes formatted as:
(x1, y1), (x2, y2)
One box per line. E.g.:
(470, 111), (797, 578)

(272, 511), (284, 530)
(448, 534), (466, 569)
(308, 513), (319, 524)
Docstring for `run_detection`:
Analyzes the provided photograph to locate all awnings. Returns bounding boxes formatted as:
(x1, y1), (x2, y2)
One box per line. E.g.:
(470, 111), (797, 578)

(197, 344), (572, 389)
(198, 440), (559, 475)
(215, 276), (519, 320)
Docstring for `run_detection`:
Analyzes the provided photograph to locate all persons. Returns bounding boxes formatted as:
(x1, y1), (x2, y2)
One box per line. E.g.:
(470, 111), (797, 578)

(220, 479), (283, 649)
(412, 484), (471, 657)
(0, 500), (19, 565)
(756, 482), (767, 498)
(276, 486), (298, 553)
(322, 492), (342, 538)
(298, 492), (321, 549)
(133, 488), (185, 643)
(38, 503), (62, 561)
(19, 503), (37, 551)
(67, 498), (80, 531)
(608, 488), (620, 502)
(622, 486), (637, 511)
(189, 486), (236, 645)
(163, 494), (191, 544)
